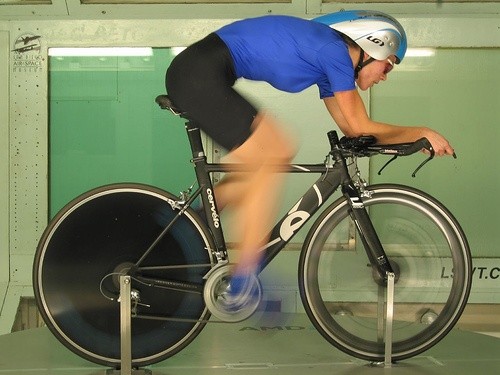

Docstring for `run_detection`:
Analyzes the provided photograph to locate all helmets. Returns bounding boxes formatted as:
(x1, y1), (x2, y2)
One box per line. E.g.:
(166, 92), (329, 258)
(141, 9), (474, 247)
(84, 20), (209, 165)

(311, 10), (407, 65)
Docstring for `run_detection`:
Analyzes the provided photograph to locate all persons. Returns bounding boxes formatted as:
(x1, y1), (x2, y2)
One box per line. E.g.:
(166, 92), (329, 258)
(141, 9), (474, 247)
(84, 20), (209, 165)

(164, 10), (452, 309)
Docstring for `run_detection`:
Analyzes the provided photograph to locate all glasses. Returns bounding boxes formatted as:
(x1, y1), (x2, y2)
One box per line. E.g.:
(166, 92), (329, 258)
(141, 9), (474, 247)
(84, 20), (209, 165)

(383, 58), (394, 75)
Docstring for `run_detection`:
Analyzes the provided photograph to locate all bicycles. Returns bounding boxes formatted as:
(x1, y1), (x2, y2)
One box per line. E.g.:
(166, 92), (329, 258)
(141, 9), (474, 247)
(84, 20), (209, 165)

(31, 94), (474, 370)
